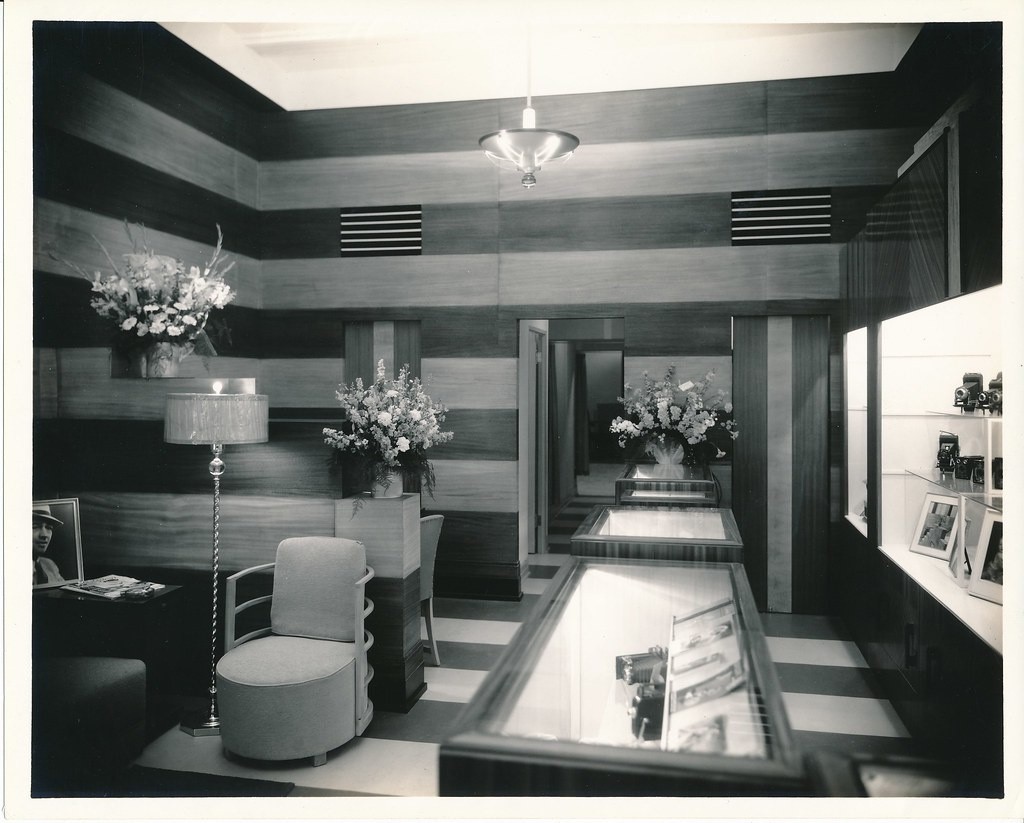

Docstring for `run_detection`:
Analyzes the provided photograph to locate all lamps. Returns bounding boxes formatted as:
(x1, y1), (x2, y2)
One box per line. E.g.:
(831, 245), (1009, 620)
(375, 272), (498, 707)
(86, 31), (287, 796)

(164, 393), (268, 736)
(478, 33), (579, 188)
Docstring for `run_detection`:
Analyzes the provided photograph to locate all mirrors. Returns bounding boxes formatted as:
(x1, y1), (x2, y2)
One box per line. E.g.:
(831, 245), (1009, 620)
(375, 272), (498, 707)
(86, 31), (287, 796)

(515, 317), (831, 613)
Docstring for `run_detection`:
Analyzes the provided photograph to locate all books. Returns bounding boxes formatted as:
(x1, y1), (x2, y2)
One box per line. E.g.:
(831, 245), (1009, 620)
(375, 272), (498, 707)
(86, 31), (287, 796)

(61, 575), (165, 599)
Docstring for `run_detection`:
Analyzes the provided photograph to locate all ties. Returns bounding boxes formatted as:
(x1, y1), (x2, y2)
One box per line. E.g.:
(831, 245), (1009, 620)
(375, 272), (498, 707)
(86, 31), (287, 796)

(35, 562), (48, 585)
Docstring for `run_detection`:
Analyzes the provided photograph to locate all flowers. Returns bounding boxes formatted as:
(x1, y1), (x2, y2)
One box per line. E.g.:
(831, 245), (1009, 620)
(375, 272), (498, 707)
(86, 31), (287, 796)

(321, 357), (454, 521)
(49, 220), (238, 377)
(607, 362), (740, 460)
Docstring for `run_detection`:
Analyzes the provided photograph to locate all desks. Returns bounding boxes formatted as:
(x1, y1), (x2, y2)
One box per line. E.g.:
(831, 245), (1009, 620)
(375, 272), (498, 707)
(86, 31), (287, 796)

(438, 557), (801, 797)
(570, 505), (742, 563)
(614, 464), (718, 505)
(46, 581), (184, 660)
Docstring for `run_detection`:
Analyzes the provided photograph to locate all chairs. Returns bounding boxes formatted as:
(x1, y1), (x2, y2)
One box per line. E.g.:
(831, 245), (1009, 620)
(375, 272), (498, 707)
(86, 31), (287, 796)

(420, 515), (444, 666)
(213, 536), (374, 770)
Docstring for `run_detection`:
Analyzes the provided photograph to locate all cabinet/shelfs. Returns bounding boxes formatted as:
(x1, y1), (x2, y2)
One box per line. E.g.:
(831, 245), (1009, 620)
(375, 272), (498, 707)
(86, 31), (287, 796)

(905, 409), (1003, 513)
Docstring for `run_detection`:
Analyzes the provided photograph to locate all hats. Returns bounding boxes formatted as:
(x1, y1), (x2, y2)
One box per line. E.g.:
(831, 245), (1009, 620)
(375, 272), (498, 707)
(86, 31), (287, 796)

(34, 505), (64, 528)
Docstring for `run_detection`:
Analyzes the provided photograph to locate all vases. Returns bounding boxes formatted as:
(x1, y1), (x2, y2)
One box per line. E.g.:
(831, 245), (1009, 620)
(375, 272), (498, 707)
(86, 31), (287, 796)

(146, 340), (173, 379)
(650, 438), (691, 464)
(369, 470), (404, 498)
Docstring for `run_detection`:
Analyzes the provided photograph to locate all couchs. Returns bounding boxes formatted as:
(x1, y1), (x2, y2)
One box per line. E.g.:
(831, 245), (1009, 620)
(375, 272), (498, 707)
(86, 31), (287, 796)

(31, 654), (148, 798)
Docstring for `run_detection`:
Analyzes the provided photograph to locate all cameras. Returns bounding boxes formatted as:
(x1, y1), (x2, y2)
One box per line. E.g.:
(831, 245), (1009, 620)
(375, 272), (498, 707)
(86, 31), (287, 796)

(615, 644), (667, 741)
(937, 431), (1003, 490)
(954, 372), (1003, 416)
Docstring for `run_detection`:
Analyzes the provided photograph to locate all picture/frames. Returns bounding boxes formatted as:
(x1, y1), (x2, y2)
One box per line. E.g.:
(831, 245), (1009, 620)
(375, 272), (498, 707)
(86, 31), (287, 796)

(32, 498), (85, 591)
(956, 495), (964, 586)
(967, 508), (1003, 606)
(908, 493), (967, 562)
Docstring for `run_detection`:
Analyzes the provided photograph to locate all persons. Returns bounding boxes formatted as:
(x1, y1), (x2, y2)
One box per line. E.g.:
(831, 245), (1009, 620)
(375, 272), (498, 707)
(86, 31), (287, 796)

(32, 505), (66, 585)
